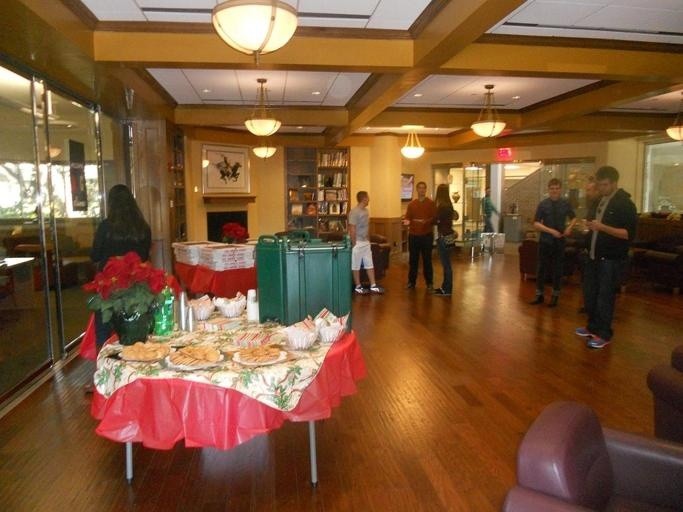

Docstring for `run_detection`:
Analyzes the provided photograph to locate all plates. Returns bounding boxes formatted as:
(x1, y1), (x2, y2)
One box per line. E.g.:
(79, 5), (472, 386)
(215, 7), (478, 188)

(231, 349), (288, 365)
(117, 347), (178, 368)
(165, 348), (224, 371)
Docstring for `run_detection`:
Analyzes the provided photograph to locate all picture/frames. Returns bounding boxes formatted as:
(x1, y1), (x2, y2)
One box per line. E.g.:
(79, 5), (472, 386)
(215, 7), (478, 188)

(201, 143), (251, 194)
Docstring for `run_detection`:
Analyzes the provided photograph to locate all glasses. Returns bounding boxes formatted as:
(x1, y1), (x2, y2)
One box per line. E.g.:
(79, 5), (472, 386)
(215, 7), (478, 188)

(597, 201), (604, 213)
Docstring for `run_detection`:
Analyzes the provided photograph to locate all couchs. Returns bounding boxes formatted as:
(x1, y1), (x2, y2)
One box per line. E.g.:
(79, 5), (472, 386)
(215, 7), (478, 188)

(319, 231), (390, 280)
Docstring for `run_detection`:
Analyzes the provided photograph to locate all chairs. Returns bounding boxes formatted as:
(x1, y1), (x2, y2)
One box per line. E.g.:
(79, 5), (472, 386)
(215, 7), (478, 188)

(646, 344), (683, 443)
(502, 398), (683, 511)
(519, 236), (682, 293)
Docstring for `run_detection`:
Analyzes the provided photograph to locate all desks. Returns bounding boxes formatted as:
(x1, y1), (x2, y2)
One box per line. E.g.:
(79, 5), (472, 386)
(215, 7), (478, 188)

(175, 262), (257, 298)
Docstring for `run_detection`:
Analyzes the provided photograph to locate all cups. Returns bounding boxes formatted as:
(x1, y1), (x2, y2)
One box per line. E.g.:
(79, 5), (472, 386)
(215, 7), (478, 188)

(247, 288), (260, 323)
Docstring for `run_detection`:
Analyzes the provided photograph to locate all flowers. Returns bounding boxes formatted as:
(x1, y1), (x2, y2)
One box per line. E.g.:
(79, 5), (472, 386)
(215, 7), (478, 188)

(222, 223), (249, 244)
(82, 251), (181, 324)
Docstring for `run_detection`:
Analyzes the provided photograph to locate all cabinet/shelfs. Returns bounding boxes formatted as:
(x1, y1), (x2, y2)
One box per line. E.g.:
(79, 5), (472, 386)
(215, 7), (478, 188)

(284, 145), (351, 238)
(166, 119), (188, 242)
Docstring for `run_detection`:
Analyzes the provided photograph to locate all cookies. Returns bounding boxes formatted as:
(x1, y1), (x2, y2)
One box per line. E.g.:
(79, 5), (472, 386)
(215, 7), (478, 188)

(169, 346), (218, 366)
(121, 341), (171, 360)
(240, 346), (280, 363)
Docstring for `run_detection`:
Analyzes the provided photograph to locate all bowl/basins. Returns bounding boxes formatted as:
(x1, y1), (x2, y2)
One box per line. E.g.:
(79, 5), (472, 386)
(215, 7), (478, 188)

(286, 328), (318, 350)
(216, 300), (248, 318)
(318, 326), (347, 344)
(189, 303), (216, 321)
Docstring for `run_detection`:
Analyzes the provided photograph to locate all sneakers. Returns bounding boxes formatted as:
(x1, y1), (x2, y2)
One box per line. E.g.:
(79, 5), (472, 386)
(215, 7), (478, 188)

(370, 287), (384, 293)
(575, 327), (596, 338)
(587, 335), (610, 348)
(354, 287), (368, 294)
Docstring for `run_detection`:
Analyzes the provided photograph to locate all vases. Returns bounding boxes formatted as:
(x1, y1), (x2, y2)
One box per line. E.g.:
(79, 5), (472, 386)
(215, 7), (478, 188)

(112, 306), (151, 346)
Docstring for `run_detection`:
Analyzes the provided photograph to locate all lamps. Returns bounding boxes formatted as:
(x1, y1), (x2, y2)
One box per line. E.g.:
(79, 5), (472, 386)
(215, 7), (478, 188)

(401, 129), (424, 158)
(470, 85), (507, 137)
(250, 137), (277, 160)
(212, 0), (299, 66)
(244, 78), (282, 137)
(666, 92), (683, 140)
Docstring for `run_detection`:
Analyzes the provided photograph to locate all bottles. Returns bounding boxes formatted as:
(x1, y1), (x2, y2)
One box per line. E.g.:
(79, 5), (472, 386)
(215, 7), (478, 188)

(188, 306), (195, 332)
(179, 291), (188, 329)
(153, 285), (175, 335)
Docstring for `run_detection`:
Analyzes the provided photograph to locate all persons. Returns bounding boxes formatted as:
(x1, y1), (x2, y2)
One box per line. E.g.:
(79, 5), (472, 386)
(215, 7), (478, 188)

(215, 156), (233, 174)
(480, 186), (502, 233)
(586, 182), (601, 204)
(572, 164), (638, 351)
(80, 182), (153, 393)
(527, 178), (577, 307)
(400, 181), (439, 291)
(412, 182), (459, 297)
(347, 190), (385, 295)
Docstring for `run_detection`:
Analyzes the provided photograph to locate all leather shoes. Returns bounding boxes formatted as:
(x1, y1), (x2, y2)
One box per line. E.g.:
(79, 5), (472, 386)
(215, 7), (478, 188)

(578, 305), (585, 312)
(434, 288), (444, 291)
(548, 295), (558, 307)
(528, 295), (544, 305)
(427, 283), (432, 290)
(434, 290), (451, 296)
(83, 384), (92, 393)
(408, 283), (416, 289)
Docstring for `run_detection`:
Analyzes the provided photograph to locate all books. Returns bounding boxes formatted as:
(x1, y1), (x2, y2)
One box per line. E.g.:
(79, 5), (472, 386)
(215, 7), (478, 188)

(287, 150), (349, 232)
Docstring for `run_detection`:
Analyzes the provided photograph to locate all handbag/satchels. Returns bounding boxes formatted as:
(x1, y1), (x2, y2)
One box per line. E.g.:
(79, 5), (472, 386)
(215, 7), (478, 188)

(440, 233), (456, 249)
